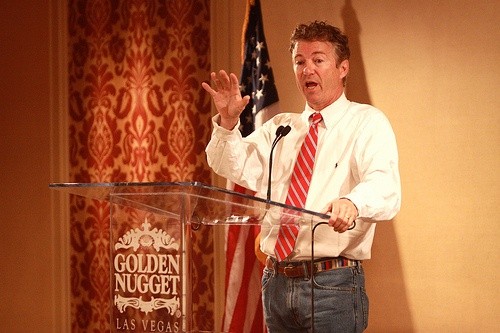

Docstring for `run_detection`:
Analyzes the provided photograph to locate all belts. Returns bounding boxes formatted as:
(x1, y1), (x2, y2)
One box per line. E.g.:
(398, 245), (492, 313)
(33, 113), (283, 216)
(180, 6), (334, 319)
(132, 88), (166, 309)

(265, 257), (362, 278)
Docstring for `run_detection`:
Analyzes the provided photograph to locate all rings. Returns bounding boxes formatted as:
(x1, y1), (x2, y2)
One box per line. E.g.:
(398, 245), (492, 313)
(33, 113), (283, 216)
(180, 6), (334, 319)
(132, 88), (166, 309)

(345, 215), (350, 220)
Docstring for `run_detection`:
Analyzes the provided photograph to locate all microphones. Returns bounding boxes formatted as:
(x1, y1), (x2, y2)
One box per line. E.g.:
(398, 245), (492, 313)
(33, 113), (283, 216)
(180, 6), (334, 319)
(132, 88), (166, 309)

(266, 125), (292, 209)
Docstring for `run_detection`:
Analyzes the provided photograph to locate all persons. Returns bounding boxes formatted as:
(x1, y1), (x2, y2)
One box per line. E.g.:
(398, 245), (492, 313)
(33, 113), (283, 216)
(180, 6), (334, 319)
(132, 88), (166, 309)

(200, 20), (402, 333)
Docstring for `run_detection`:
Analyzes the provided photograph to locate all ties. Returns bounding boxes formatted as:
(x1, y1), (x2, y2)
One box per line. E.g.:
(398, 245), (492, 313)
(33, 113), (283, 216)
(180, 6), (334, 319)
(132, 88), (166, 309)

(274, 113), (323, 263)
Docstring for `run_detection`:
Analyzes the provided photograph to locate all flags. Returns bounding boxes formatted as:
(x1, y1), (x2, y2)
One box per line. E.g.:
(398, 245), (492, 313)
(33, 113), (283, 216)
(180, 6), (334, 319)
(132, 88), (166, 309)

(212, 1), (282, 333)
